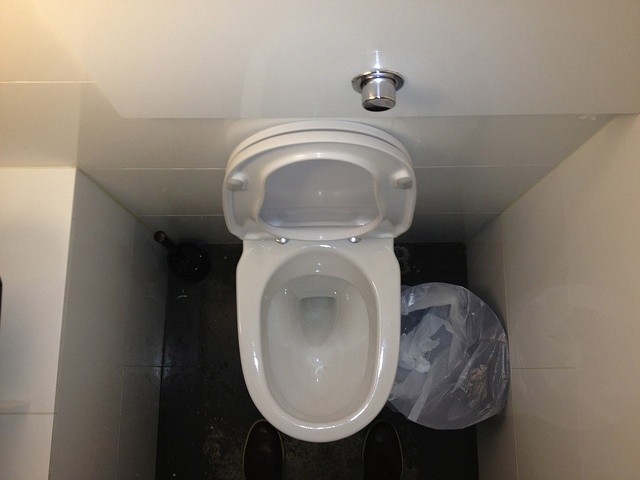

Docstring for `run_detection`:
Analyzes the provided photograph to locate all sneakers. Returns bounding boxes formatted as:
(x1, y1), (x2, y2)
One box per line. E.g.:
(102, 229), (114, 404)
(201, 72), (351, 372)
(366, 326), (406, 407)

(242, 420), (284, 479)
(362, 421), (403, 479)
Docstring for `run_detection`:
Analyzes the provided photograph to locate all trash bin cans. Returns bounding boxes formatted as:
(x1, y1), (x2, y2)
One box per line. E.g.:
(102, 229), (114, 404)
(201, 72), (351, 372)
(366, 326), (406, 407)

(387, 282), (509, 432)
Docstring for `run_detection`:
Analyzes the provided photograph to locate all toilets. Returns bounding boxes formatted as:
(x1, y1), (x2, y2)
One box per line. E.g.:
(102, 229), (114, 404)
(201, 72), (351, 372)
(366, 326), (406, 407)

(220, 118), (419, 445)
